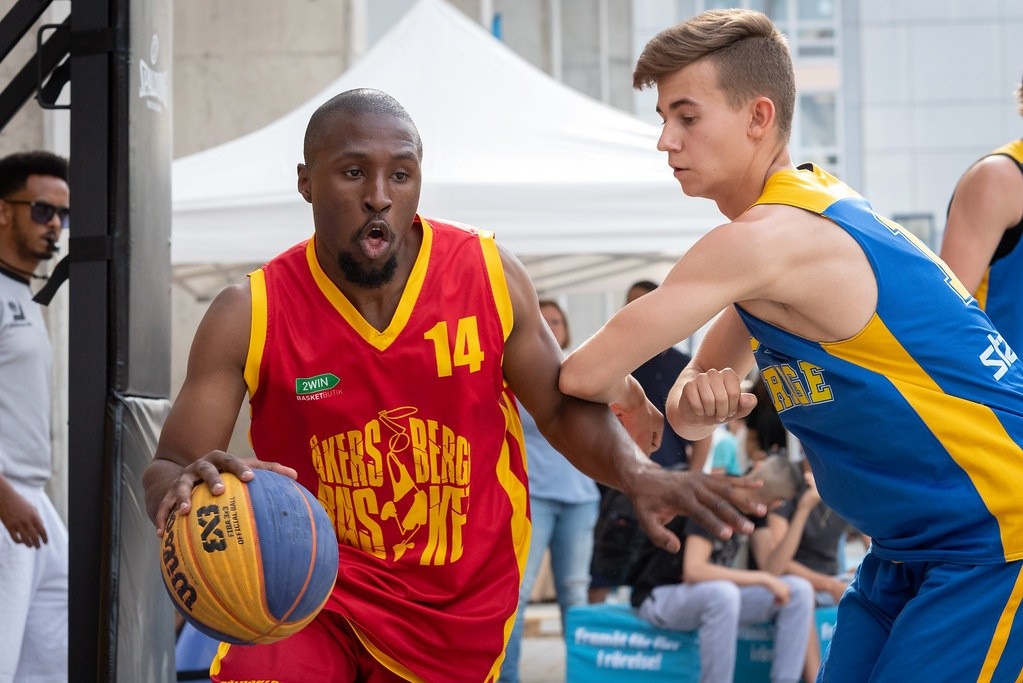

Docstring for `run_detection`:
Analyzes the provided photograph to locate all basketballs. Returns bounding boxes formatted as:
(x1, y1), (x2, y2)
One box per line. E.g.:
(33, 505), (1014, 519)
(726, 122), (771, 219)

(159, 466), (341, 648)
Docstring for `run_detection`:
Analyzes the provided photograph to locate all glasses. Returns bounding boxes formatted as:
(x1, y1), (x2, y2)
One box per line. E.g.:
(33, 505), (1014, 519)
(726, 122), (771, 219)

(5, 199), (69, 230)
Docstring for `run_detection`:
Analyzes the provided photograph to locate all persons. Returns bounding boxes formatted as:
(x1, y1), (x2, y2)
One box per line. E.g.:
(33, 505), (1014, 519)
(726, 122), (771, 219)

(503, 280), (874, 683)
(0, 152), (69, 683)
(140, 88), (766, 683)
(938, 82), (1023, 365)
(558, 7), (1023, 683)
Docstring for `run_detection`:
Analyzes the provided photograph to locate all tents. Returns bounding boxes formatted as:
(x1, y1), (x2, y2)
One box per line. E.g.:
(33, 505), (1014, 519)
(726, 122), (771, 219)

(43, 0), (733, 533)
